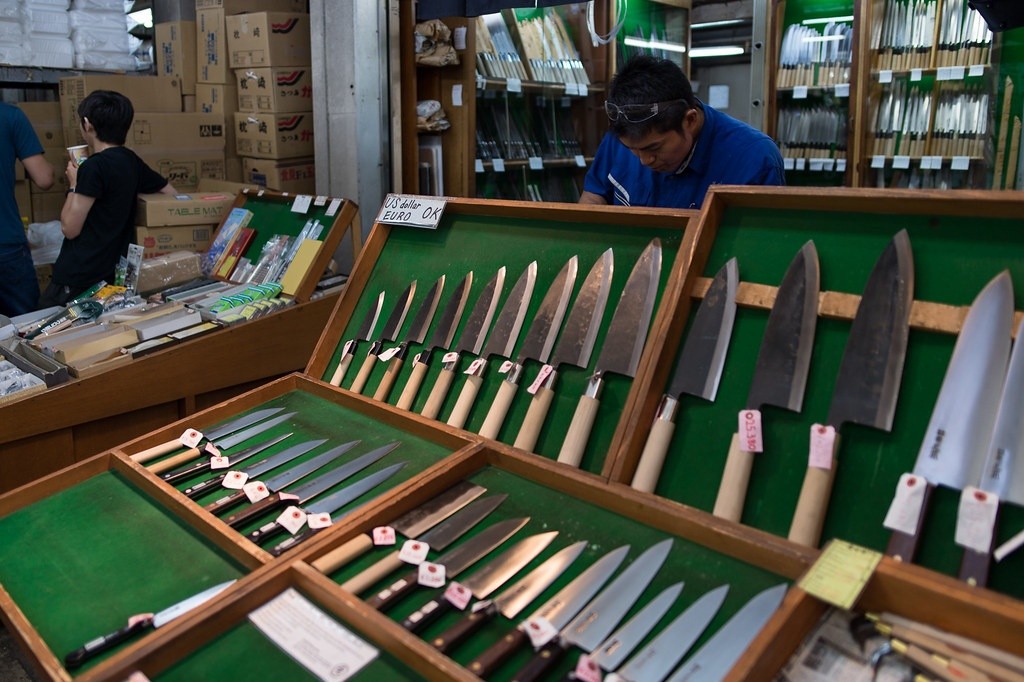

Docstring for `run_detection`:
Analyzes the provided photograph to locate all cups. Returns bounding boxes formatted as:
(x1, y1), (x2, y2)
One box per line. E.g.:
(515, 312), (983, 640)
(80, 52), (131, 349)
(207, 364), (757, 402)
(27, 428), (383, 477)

(67, 145), (90, 168)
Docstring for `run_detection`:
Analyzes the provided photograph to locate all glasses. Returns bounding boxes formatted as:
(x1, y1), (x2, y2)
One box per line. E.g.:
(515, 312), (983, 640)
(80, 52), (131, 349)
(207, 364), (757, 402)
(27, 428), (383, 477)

(604, 99), (688, 123)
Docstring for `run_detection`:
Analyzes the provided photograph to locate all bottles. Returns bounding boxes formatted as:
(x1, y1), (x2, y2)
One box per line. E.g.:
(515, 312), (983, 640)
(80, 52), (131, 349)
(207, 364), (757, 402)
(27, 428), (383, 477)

(20, 216), (29, 237)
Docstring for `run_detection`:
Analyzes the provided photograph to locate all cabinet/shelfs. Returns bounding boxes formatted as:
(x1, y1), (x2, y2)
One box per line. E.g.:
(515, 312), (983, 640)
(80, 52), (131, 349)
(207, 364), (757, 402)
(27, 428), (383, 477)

(609, 0), (690, 82)
(762, 1), (1001, 188)
(402, 0), (606, 201)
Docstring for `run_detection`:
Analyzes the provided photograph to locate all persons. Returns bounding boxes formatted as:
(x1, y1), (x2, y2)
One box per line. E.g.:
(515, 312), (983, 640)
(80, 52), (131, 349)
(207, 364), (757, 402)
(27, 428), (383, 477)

(46, 89), (176, 308)
(578, 54), (784, 208)
(0, 101), (55, 318)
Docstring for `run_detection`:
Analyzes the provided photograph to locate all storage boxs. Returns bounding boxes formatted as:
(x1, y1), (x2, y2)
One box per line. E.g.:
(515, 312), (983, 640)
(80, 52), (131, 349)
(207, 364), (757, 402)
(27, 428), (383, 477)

(1, 0), (313, 291)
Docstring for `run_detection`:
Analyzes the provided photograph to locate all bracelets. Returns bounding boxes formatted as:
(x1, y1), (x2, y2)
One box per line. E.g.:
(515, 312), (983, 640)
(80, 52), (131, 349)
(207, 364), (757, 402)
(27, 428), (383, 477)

(65, 188), (74, 198)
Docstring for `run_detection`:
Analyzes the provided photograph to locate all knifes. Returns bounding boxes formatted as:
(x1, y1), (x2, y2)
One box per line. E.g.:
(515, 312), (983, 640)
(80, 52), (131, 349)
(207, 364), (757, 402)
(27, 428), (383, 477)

(775, 20), (853, 87)
(470, 10), (669, 203)
(63, 578), (239, 670)
(629, 226), (1024, 588)
(776, 102), (846, 159)
(873, 77), (933, 157)
(876, 1), (993, 70)
(930, 79), (988, 157)
(129, 408), (409, 556)
(311, 480), (788, 681)
(327, 237), (663, 469)
